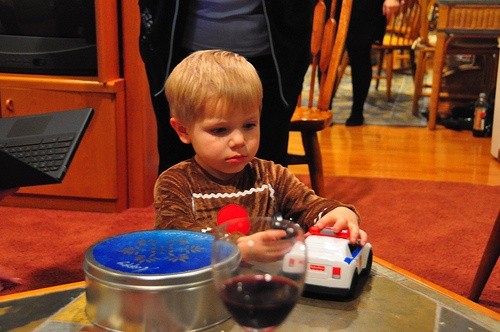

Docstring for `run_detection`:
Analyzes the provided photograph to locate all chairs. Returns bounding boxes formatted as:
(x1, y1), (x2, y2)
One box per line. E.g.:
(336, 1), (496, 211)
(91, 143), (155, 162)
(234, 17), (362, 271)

(335, 0), (499, 129)
(287, 0), (352, 198)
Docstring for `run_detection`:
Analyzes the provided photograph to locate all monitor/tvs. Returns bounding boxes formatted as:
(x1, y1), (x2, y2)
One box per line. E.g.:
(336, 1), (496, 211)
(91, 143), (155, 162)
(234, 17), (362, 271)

(0, 0), (98, 77)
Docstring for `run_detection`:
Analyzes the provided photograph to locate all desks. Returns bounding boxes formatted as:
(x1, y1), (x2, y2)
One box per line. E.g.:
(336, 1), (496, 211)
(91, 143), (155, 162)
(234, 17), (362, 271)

(0, 255), (500, 332)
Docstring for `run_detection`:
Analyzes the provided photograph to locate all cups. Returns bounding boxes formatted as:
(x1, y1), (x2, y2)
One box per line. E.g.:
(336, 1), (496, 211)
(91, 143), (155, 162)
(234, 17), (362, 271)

(211, 216), (308, 332)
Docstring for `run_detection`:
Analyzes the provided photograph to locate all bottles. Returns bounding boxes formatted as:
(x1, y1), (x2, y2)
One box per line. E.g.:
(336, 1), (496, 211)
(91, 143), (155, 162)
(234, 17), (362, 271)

(472, 93), (489, 137)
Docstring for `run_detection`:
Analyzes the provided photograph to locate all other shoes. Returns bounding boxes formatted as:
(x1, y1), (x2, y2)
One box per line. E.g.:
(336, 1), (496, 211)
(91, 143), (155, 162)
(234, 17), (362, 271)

(346, 115), (365, 126)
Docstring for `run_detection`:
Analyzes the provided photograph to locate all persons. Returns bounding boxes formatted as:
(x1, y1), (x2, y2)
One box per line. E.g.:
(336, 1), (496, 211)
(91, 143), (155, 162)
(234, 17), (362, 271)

(138, 0), (317, 181)
(307, 0), (403, 127)
(154, 49), (369, 264)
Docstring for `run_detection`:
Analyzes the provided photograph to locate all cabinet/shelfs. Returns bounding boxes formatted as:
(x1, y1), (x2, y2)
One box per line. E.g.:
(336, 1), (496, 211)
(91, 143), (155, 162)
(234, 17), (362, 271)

(0, 0), (156, 212)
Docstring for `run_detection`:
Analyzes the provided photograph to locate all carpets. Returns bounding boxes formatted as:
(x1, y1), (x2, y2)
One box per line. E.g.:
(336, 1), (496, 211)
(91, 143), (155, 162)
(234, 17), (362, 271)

(0, 171), (500, 317)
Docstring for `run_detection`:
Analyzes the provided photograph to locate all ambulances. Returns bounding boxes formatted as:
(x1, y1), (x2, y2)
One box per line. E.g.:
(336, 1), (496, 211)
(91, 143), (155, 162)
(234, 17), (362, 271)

(283, 225), (374, 300)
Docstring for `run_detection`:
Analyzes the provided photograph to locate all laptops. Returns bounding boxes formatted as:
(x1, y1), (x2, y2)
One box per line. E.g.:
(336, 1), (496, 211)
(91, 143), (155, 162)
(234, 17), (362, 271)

(0, 108), (95, 189)
